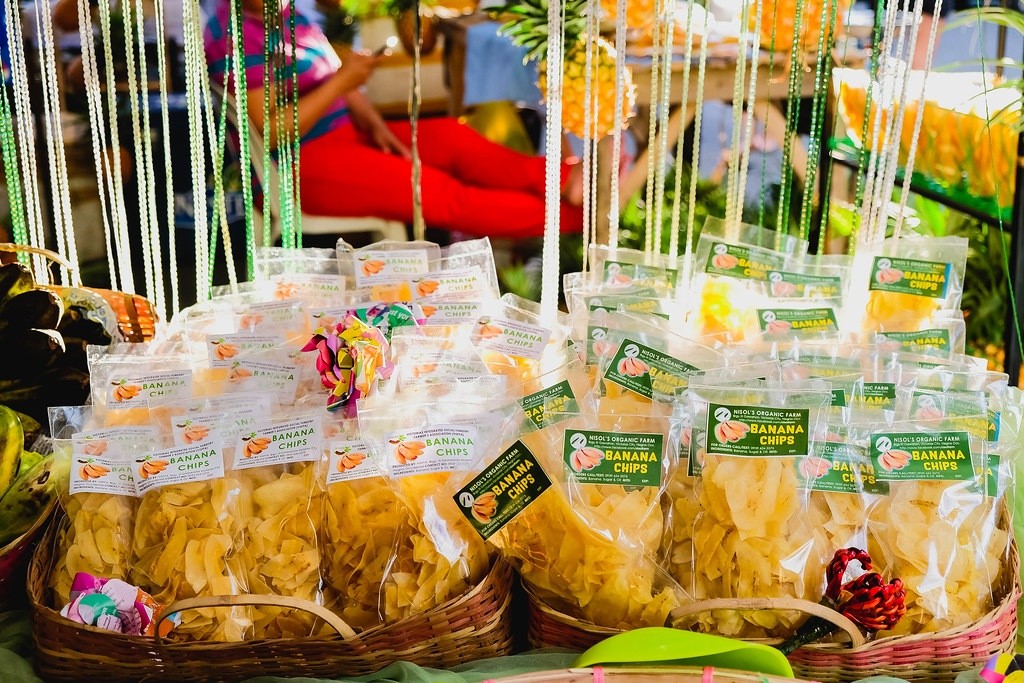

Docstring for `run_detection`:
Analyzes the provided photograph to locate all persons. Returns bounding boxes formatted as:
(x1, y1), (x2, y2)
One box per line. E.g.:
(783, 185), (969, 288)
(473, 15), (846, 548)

(200, 0), (625, 238)
(52, 0), (213, 87)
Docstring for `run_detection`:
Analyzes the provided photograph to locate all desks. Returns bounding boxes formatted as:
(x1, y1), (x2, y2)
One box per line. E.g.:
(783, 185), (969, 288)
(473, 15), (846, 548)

(435, 12), (874, 255)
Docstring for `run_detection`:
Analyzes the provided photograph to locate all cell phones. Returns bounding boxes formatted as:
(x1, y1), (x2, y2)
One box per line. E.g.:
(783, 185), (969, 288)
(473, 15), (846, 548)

(372, 37), (398, 58)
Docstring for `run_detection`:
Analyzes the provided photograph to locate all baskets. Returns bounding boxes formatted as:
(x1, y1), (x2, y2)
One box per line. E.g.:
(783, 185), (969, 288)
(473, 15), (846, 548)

(0, 241), (161, 342)
(23, 471), (515, 683)
(526, 493), (1023, 683)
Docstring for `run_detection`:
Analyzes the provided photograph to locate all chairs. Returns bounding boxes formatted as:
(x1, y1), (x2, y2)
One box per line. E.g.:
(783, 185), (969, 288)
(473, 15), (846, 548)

(178, 55), (407, 257)
